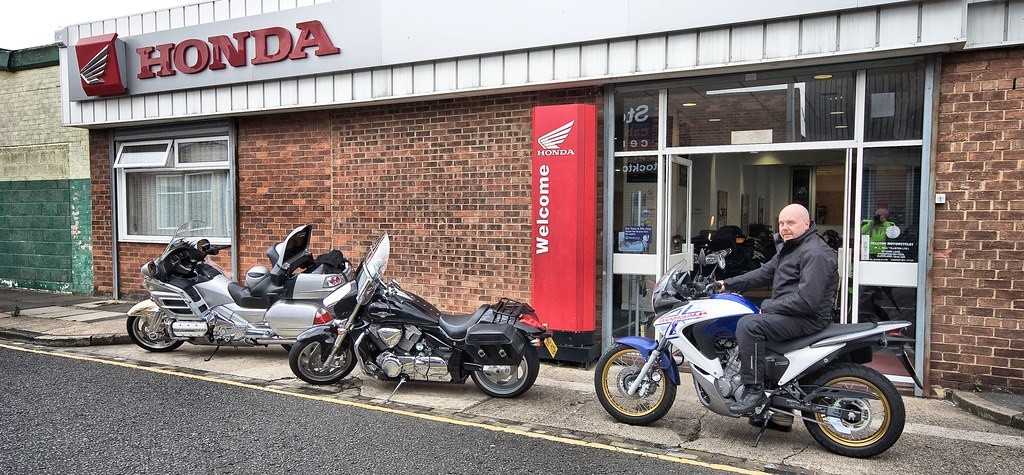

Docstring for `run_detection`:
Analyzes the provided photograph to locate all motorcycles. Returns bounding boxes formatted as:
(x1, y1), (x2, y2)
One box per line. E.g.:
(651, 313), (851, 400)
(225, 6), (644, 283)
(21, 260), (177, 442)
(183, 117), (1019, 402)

(592, 248), (926, 460)
(287, 230), (558, 406)
(123, 218), (357, 362)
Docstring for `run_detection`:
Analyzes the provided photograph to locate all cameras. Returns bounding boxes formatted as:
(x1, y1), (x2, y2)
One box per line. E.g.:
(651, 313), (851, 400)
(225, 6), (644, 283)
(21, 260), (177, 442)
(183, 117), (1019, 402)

(873, 215), (881, 225)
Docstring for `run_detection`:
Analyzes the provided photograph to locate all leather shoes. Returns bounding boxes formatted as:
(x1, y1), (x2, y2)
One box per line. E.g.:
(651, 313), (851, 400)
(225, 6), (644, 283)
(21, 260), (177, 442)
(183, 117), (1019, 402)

(729, 382), (767, 414)
(750, 414), (792, 432)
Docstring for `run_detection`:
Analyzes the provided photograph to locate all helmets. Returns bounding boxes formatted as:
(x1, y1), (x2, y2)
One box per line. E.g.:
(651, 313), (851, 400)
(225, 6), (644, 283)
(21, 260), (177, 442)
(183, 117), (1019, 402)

(246, 266), (269, 291)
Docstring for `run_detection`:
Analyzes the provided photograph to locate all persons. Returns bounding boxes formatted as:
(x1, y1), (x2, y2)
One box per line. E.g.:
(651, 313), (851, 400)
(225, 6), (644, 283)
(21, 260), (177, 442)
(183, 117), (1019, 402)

(713, 203), (839, 432)
(860, 209), (895, 260)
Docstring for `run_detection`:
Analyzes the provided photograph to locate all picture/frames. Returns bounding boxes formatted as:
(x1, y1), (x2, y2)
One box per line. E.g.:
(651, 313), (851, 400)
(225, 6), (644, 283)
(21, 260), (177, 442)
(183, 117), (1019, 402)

(718, 188), (729, 231)
(758, 196), (765, 225)
(740, 194), (750, 236)
